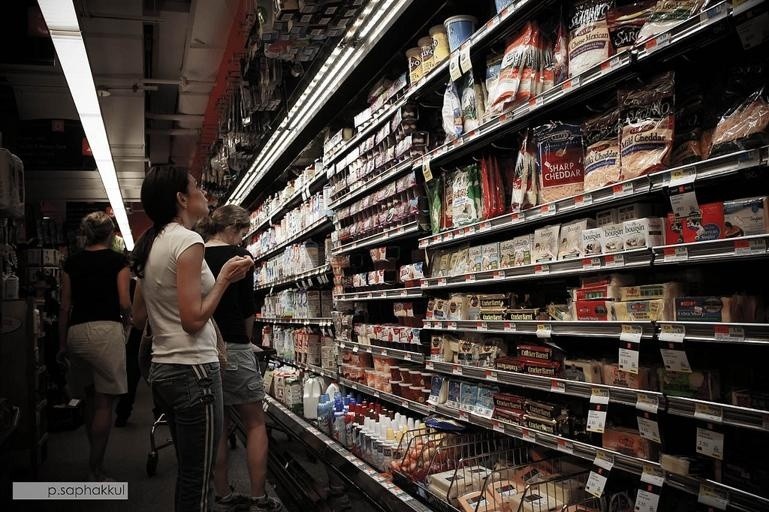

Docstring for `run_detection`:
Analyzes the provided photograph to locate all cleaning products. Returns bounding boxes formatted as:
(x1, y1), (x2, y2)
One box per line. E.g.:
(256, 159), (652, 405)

(318, 390), (420, 474)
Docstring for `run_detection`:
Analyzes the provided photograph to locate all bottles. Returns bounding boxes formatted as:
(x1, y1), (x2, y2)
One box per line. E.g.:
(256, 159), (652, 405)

(313, 390), (437, 476)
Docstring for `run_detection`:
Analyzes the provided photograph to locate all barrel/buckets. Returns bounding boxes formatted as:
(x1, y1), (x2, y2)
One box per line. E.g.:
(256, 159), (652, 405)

(302, 375), (322, 421)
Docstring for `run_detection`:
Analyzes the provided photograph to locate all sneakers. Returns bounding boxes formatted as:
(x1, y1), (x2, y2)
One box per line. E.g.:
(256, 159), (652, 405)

(114, 415), (128, 428)
(213, 491), (248, 511)
(249, 494), (282, 511)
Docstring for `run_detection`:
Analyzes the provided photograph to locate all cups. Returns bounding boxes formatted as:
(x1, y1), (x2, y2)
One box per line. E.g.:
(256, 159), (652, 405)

(403, 13), (477, 80)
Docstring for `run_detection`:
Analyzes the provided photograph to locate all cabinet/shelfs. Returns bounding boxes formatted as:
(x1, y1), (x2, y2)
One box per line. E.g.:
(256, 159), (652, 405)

(0, 0), (768, 512)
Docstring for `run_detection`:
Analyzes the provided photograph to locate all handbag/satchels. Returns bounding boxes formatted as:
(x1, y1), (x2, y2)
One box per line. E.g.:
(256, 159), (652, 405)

(41, 357), (92, 478)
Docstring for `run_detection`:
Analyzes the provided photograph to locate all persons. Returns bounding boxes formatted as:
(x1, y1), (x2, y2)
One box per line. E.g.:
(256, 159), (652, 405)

(55, 209), (131, 482)
(196, 203), (282, 511)
(18, 270), (60, 348)
(129, 163), (256, 511)
(114, 275), (170, 427)
